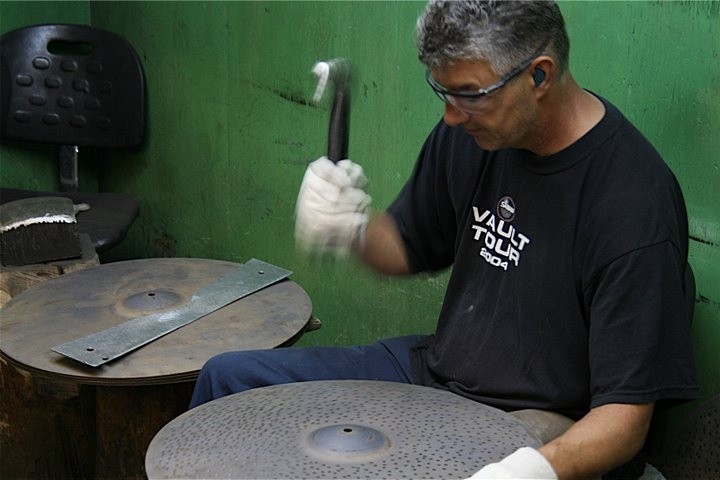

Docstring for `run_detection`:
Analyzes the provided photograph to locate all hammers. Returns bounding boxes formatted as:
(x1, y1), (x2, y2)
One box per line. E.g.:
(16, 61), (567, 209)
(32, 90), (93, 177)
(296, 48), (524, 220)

(313, 61), (360, 165)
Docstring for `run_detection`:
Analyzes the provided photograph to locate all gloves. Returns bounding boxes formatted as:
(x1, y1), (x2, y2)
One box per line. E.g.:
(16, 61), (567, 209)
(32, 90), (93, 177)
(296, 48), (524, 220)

(467, 446), (558, 480)
(295, 156), (372, 254)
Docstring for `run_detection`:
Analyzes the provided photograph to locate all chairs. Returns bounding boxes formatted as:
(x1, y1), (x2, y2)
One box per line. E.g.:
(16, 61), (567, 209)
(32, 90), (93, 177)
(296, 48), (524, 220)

(0, 24), (148, 255)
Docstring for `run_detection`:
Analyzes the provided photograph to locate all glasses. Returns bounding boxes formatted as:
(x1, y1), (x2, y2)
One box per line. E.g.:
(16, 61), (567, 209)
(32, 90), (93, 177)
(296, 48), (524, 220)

(426, 41), (549, 114)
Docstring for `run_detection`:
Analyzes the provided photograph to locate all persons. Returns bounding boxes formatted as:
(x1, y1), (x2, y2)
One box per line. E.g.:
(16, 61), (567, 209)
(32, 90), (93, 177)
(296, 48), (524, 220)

(190, 0), (700, 480)
